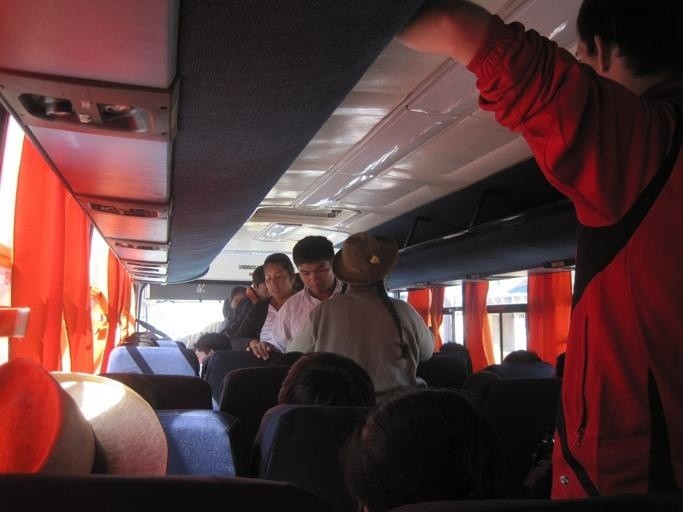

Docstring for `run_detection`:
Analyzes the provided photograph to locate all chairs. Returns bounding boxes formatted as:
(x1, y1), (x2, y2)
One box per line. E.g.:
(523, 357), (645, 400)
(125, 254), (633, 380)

(153, 403), (382, 512)
(416, 353), (564, 512)
(1, 477), (332, 512)
(98, 337), (305, 409)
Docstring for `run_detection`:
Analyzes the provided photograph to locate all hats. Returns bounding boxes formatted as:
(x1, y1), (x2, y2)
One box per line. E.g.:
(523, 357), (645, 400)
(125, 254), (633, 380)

(331, 231), (401, 288)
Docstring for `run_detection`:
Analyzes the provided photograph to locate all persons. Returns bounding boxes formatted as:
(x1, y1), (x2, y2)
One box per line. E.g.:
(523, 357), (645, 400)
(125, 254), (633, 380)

(396, 0), (683, 511)
(0, 358), (169, 476)
